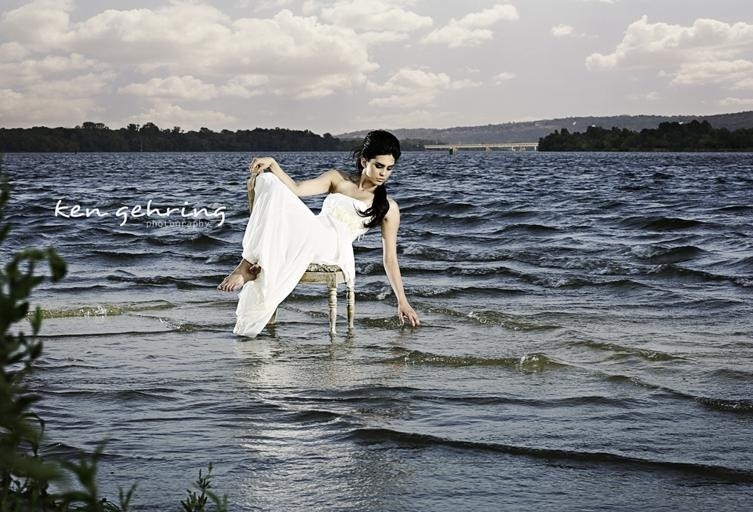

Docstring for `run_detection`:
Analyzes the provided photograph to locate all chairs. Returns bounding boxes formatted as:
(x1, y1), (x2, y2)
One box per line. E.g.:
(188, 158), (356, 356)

(247, 165), (356, 334)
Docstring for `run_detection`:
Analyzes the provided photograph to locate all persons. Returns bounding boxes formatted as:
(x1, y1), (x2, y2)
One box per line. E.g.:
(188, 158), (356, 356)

(218, 129), (421, 339)
